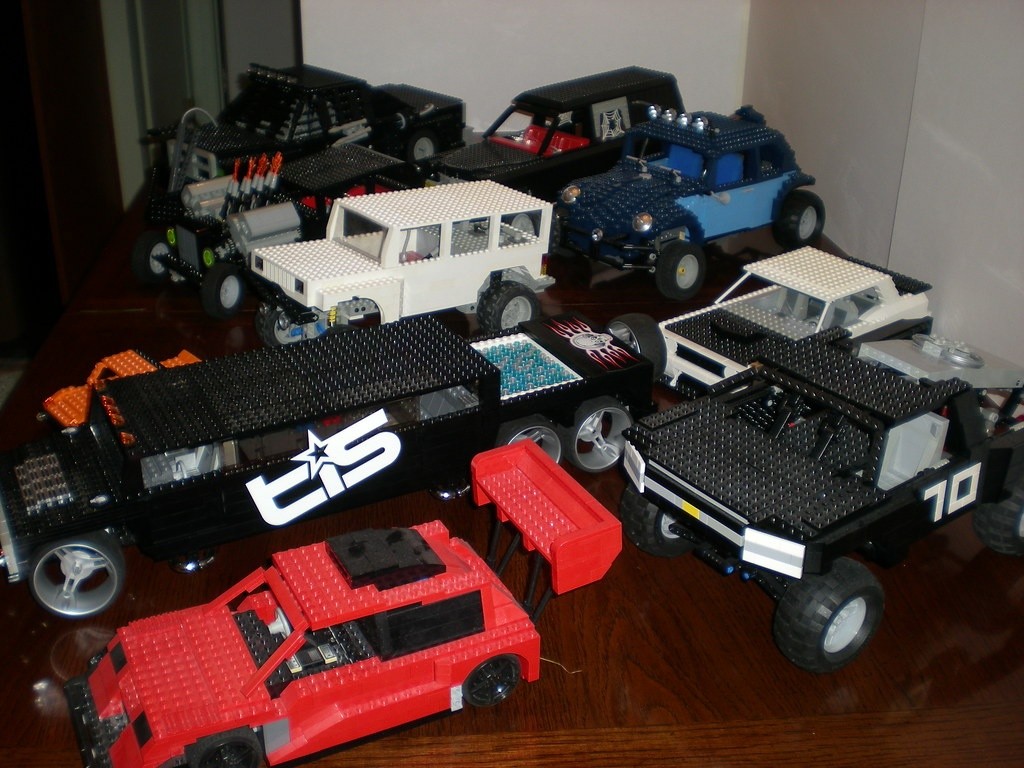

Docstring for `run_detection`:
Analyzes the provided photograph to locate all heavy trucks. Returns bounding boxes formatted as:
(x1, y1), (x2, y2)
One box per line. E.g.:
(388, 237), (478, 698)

(0, 313), (648, 621)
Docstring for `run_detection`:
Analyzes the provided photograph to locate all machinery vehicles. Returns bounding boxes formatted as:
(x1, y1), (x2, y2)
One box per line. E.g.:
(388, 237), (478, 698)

(609, 318), (1023, 673)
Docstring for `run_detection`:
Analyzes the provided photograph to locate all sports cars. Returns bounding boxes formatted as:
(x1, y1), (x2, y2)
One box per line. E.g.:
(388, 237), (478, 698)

(59, 439), (625, 768)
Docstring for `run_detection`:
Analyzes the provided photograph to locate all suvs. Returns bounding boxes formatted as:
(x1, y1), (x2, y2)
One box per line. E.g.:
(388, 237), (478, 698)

(434, 65), (688, 199)
(137, 63), (468, 252)
(550, 100), (827, 305)
(648, 244), (934, 400)
(252, 180), (555, 346)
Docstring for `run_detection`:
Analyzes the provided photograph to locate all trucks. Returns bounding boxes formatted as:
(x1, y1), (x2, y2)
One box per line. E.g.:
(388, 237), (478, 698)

(126, 142), (425, 321)
